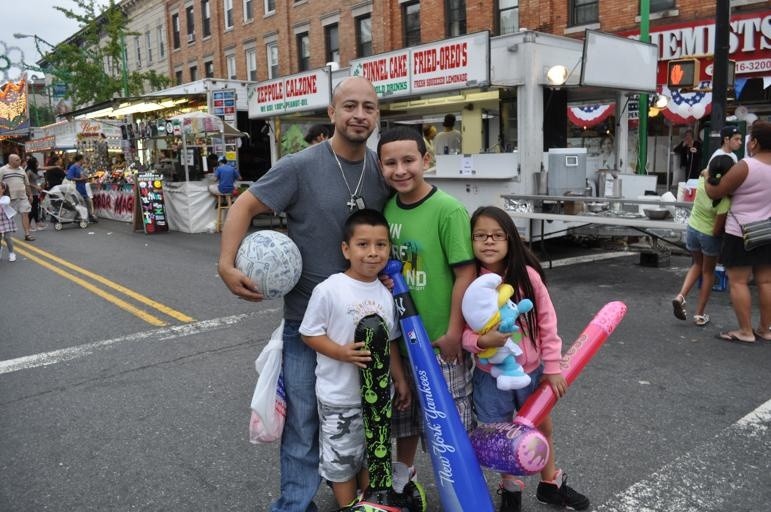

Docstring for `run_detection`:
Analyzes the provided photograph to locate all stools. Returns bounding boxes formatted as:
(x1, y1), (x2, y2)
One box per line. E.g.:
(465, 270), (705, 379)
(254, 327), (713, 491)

(215, 192), (233, 235)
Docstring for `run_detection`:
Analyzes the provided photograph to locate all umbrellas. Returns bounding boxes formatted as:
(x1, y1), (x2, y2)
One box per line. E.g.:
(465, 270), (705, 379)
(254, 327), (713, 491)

(154, 109), (246, 137)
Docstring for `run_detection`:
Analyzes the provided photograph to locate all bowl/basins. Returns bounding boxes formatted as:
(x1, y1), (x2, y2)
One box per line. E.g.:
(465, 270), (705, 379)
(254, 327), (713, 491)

(587, 203), (608, 212)
(643, 209), (669, 220)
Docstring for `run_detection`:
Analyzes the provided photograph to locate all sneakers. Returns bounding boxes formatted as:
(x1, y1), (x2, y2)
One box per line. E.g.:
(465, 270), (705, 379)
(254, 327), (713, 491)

(0, 246), (3, 260)
(497, 480), (523, 512)
(28, 227), (37, 232)
(37, 224), (48, 231)
(536, 468), (589, 510)
(9, 252), (16, 263)
(390, 462), (418, 493)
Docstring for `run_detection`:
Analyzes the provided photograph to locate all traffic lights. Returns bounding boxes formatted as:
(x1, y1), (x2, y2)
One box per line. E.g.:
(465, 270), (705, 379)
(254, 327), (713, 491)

(667, 59), (701, 91)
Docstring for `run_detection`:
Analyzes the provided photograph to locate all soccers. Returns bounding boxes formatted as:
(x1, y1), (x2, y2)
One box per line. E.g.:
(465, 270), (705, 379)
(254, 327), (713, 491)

(234, 229), (303, 301)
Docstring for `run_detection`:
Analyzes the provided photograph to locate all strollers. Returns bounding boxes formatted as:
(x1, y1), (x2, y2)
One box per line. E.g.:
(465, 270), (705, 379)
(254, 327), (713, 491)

(37, 184), (88, 230)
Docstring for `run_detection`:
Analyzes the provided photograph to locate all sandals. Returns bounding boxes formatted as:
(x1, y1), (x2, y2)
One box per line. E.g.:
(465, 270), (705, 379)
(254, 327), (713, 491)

(672, 294), (688, 320)
(25, 234), (35, 240)
(694, 313), (710, 326)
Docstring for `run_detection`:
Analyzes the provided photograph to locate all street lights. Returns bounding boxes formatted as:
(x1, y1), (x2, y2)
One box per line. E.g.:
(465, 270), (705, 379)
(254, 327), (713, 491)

(11, 32), (55, 51)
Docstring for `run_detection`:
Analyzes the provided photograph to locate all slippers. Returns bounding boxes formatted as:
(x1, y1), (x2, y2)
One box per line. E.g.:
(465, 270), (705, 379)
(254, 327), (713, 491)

(753, 329), (771, 341)
(715, 331), (757, 343)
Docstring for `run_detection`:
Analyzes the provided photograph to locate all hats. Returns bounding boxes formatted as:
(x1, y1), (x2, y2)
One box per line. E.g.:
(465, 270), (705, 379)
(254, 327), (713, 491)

(720, 125), (741, 145)
(218, 156), (227, 162)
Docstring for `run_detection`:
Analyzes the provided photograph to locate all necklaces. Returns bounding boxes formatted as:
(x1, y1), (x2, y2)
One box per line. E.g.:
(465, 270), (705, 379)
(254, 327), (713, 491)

(330, 137), (367, 212)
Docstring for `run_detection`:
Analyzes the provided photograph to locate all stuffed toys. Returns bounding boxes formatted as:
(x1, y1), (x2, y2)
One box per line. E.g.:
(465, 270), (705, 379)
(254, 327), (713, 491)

(458, 274), (533, 389)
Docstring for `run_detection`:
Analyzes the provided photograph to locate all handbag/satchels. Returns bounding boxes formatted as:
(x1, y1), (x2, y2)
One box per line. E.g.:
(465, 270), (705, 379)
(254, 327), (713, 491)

(740, 216), (771, 251)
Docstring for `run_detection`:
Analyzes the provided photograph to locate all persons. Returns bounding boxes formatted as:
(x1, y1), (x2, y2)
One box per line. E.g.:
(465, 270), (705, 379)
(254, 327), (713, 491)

(0, 153), (35, 240)
(68, 156), (97, 222)
(457, 207), (590, 512)
(209, 156), (242, 209)
(217, 77), (397, 512)
(23, 157), (48, 232)
(706, 126), (742, 169)
(308, 124), (328, 147)
(423, 124), (437, 157)
(0, 181), (17, 262)
(299, 209), (410, 512)
(21, 153), (40, 173)
(377, 126), (475, 512)
(674, 131), (702, 185)
(44, 160), (66, 189)
(432, 113), (460, 155)
(672, 155), (735, 327)
(699, 119), (771, 344)
